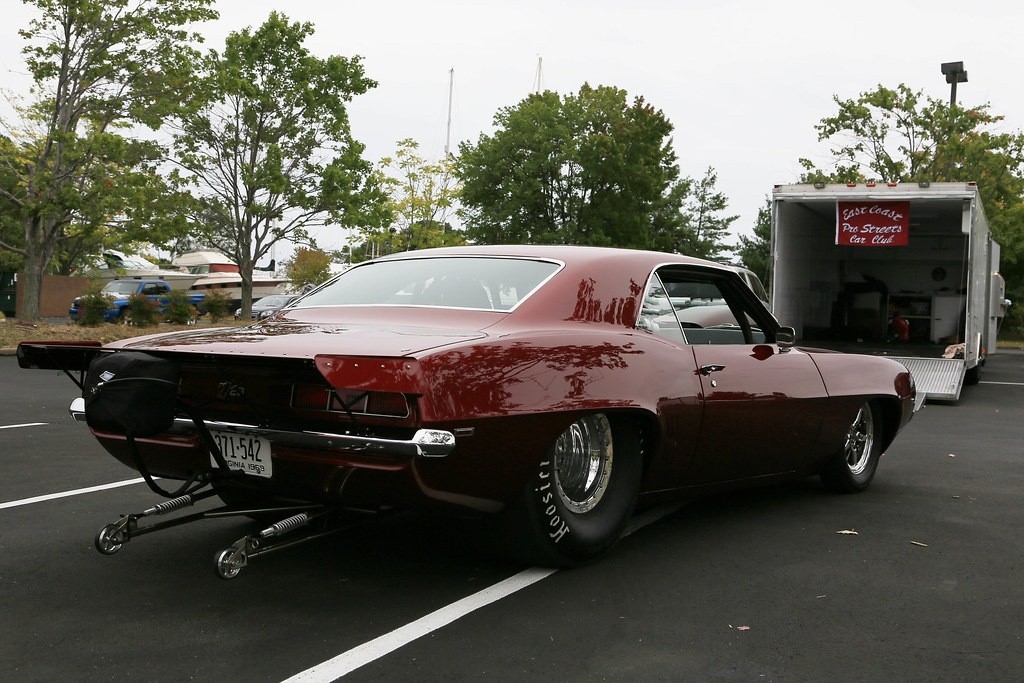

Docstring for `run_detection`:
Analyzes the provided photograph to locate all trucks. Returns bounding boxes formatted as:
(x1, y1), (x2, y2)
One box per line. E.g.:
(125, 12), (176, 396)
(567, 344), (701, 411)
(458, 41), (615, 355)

(767, 180), (1013, 404)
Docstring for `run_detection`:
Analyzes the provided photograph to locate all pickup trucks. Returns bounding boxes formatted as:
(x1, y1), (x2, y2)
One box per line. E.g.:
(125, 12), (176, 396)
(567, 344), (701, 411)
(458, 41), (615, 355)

(69, 278), (207, 327)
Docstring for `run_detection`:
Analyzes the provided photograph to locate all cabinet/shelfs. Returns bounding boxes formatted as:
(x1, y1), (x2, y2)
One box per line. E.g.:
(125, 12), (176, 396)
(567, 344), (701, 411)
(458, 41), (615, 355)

(885, 292), (931, 340)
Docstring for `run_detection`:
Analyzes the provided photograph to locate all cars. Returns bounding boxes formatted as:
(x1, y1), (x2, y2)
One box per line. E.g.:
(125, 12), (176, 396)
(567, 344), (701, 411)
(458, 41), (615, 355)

(233, 295), (301, 323)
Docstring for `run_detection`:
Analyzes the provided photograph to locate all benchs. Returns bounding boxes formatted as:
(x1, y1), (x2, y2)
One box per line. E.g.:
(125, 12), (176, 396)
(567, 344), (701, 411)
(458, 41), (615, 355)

(143, 287), (158, 295)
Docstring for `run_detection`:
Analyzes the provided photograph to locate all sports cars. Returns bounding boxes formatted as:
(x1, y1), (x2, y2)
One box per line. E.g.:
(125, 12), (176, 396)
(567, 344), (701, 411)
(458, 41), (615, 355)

(19, 243), (925, 581)
